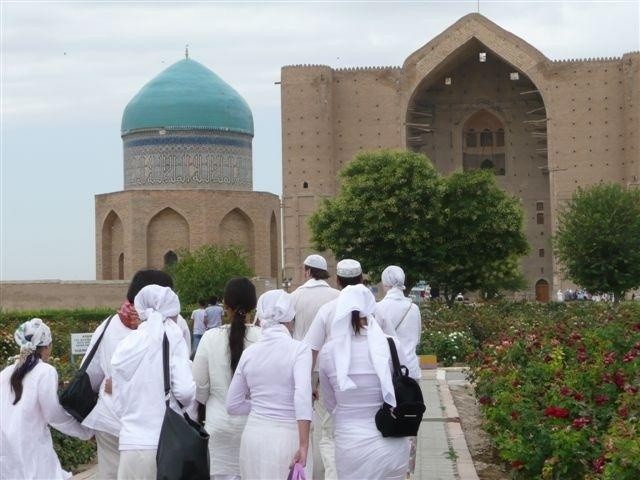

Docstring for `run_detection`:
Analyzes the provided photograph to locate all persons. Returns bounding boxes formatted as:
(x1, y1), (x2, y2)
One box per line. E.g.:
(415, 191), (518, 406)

(1, 317), (96, 479)
(79, 253), (425, 480)
(557, 287), (615, 304)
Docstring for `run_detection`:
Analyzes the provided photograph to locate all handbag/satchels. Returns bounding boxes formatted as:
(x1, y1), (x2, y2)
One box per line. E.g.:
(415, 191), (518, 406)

(375, 338), (426, 438)
(156, 408), (211, 480)
(60, 372), (96, 422)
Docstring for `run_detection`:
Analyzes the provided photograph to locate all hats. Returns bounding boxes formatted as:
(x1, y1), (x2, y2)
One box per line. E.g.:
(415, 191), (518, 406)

(336, 259), (362, 278)
(303, 254), (327, 271)
